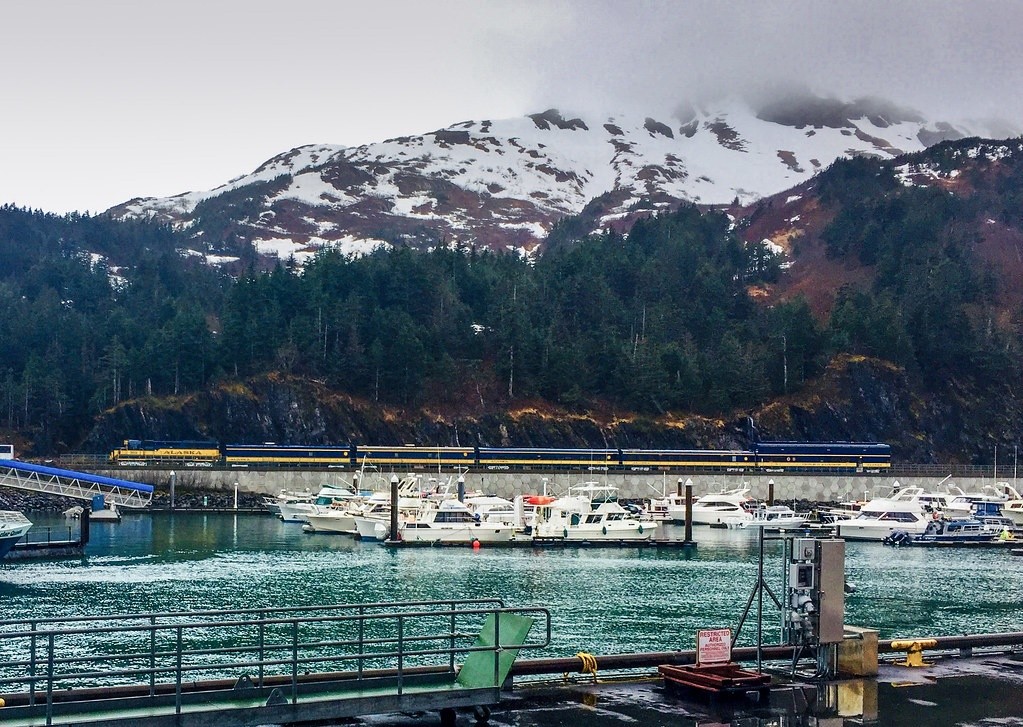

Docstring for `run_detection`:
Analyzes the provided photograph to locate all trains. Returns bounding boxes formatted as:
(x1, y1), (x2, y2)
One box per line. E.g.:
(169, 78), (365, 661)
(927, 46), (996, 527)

(108, 436), (891, 473)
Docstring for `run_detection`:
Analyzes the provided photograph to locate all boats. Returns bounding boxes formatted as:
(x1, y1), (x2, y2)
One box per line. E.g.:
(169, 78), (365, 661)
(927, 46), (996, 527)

(263, 452), (658, 549)
(641, 445), (1023, 545)
(0, 510), (33, 559)
(62, 493), (122, 523)
